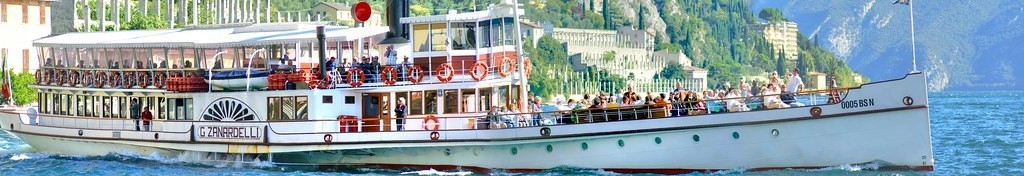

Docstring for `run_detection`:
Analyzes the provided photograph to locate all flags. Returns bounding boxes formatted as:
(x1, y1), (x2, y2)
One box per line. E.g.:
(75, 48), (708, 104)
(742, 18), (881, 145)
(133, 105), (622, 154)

(1, 57), (10, 99)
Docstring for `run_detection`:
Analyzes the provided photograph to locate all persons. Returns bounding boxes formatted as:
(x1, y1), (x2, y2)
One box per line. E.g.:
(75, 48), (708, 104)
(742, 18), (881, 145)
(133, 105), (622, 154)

(486, 69), (805, 131)
(396, 96), (406, 132)
(279, 60), (295, 72)
(326, 45), (398, 82)
(129, 98), (153, 130)
(45, 56), (193, 85)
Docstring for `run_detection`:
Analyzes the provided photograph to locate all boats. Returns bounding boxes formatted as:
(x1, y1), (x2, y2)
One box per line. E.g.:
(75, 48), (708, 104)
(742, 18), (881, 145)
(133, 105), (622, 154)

(0, 7), (934, 170)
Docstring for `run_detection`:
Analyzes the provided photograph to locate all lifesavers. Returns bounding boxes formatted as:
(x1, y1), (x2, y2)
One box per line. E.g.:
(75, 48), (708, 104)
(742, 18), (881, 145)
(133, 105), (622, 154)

(108, 72), (120, 88)
(315, 71), (330, 90)
(94, 72), (105, 88)
(35, 69), (42, 84)
(423, 115), (439, 131)
(67, 70), (78, 86)
(382, 66), (398, 85)
(347, 67), (366, 87)
(169, 72), (179, 78)
(436, 63), (454, 83)
(54, 71), (64, 86)
(153, 72), (165, 88)
(42, 69), (51, 85)
(469, 61), (488, 81)
(122, 72), (133, 88)
(499, 57), (514, 77)
(80, 71), (91, 86)
(186, 70), (197, 78)
(407, 65), (423, 84)
(524, 60), (532, 76)
(138, 71), (149, 88)
